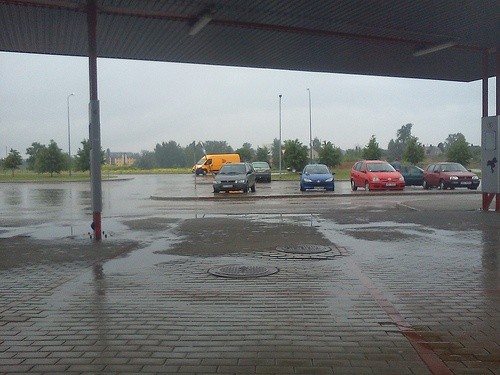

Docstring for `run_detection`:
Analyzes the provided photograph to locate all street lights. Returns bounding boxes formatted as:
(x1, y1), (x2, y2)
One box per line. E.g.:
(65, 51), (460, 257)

(307, 87), (313, 164)
(279, 95), (282, 174)
(67, 93), (75, 177)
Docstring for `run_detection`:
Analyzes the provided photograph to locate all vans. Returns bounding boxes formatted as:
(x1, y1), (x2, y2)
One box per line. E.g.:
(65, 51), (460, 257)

(192, 154), (241, 176)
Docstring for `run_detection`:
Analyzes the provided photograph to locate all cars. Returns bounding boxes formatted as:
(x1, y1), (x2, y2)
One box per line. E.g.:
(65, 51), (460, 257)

(286, 165), (301, 173)
(212, 162), (256, 192)
(350, 159), (406, 191)
(299, 164), (336, 191)
(250, 162), (271, 183)
(423, 162), (480, 189)
(398, 166), (425, 186)
